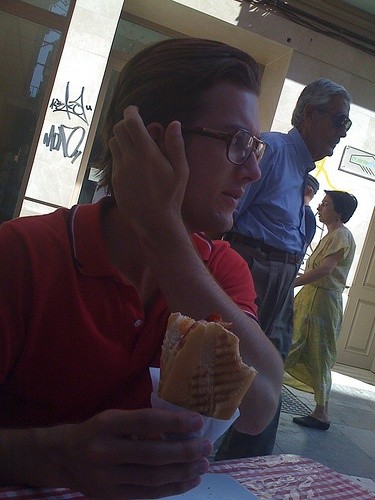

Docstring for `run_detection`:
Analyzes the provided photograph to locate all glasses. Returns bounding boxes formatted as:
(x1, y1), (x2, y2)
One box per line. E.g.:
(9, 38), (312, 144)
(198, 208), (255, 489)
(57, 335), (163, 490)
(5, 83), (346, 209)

(315, 106), (353, 132)
(319, 203), (330, 207)
(182, 127), (266, 166)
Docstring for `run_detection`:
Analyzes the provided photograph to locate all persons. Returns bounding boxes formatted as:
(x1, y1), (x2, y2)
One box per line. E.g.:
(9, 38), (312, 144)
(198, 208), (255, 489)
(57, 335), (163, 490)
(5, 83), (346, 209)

(283, 190), (358, 432)
(1, 36), (283, 500)
(204, 79), (352, 468)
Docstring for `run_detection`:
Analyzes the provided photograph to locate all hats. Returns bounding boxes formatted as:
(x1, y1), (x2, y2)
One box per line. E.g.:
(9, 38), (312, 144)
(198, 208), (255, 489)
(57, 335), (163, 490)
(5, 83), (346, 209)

(307, 174), (319, 194)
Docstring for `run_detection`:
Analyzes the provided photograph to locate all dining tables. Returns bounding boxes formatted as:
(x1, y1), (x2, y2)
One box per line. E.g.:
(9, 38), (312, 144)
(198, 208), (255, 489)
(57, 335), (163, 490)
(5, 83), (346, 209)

(0, 454), (375, 500)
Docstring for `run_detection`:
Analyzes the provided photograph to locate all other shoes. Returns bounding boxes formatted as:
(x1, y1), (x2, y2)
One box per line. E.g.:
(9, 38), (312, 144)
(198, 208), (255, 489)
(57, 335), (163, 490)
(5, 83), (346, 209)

(293, 416), (330, 430)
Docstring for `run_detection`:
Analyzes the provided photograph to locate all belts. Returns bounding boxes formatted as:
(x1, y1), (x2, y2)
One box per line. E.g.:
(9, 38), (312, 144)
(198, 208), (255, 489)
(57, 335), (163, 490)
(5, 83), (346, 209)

(223, 232), (301, 264)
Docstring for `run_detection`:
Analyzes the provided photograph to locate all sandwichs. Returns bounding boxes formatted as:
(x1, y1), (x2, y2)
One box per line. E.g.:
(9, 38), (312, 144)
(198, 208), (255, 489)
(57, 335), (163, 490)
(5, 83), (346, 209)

(156, 311), (258, 420)
